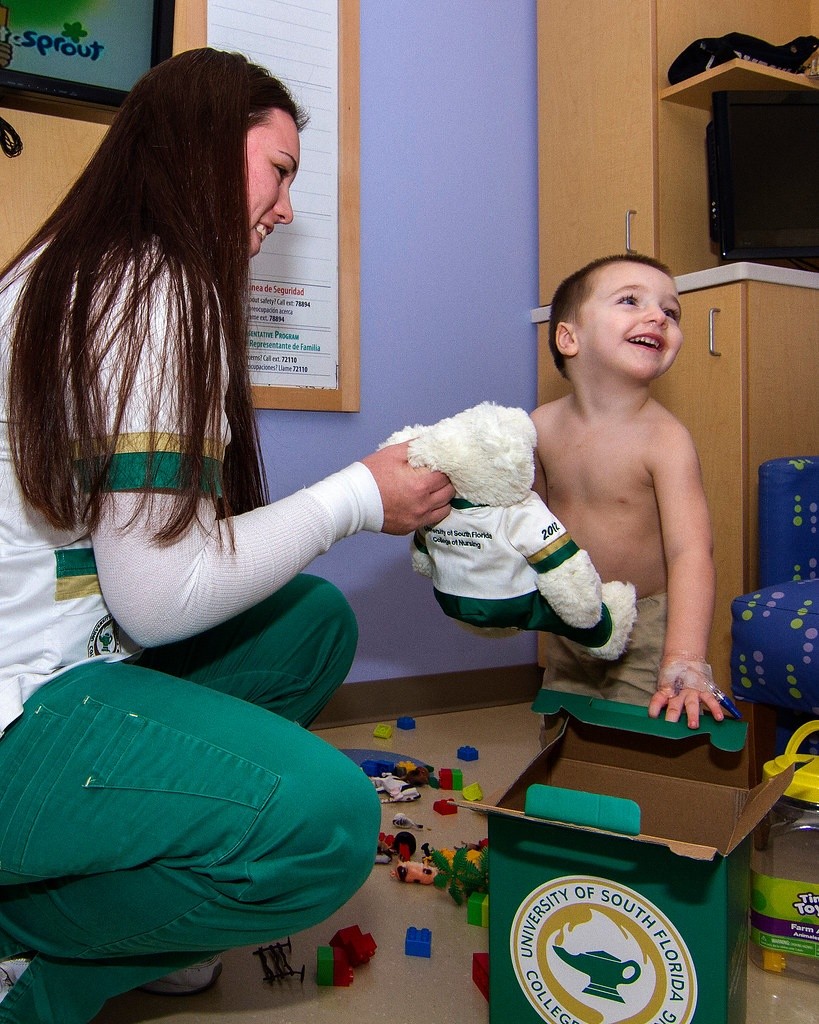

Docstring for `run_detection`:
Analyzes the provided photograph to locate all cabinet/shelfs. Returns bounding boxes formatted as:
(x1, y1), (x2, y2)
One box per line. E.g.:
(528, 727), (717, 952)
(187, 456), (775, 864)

(535, 0), (819, 701)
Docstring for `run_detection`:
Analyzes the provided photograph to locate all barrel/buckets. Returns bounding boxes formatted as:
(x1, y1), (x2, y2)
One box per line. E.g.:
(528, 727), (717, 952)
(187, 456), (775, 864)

(747, 719), (819, 983)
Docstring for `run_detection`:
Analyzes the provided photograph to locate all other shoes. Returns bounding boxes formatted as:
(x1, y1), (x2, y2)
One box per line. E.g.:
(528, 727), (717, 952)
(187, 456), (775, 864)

(134, 954), (223, 997)
(0, 957), (31, 1005)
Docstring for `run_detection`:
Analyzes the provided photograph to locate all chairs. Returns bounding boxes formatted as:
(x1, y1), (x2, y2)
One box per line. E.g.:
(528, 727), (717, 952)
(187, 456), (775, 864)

(728, 453), (819, 756)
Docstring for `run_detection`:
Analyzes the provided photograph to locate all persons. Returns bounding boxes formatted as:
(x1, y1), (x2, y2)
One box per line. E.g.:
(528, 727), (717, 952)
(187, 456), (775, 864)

(0, 39), (455, 1024)
(513, 254), (741, 736)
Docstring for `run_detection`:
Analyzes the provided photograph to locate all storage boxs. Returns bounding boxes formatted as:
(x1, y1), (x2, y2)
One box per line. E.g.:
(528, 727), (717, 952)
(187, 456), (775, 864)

(447, 691), (799, 1024)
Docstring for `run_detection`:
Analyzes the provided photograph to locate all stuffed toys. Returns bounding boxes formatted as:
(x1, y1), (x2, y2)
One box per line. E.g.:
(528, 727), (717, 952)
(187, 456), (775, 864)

(379, 401), (638, 669)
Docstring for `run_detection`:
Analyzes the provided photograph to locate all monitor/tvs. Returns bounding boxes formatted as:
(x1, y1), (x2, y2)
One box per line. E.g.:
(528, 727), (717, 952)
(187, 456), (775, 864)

(706, 88), (819, 262)
